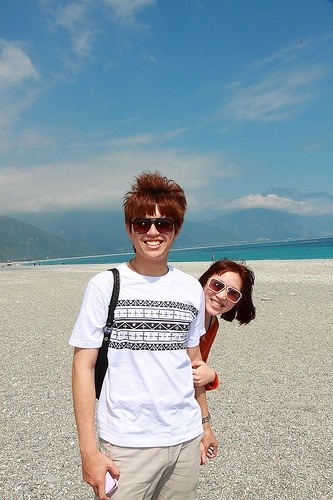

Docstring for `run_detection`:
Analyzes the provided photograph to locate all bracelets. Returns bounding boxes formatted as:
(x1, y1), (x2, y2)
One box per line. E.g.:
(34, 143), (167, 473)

(202, 412), (211, 424)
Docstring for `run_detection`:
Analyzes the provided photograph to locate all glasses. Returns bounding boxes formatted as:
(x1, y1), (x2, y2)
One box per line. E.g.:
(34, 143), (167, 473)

(207, 278), (243, 303)
(130, 218), (175, 235)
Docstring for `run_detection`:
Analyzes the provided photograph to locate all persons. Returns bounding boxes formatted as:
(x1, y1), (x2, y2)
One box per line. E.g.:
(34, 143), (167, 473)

(192, 259), (255, 458)
(69, 172), (219, 500)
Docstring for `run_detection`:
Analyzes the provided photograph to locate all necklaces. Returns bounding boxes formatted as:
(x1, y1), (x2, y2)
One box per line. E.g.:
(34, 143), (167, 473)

(130, 259), (169, 275)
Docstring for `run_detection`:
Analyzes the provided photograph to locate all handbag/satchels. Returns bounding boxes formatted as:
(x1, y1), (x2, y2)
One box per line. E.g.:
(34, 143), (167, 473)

(95, 268), (121, 400)
